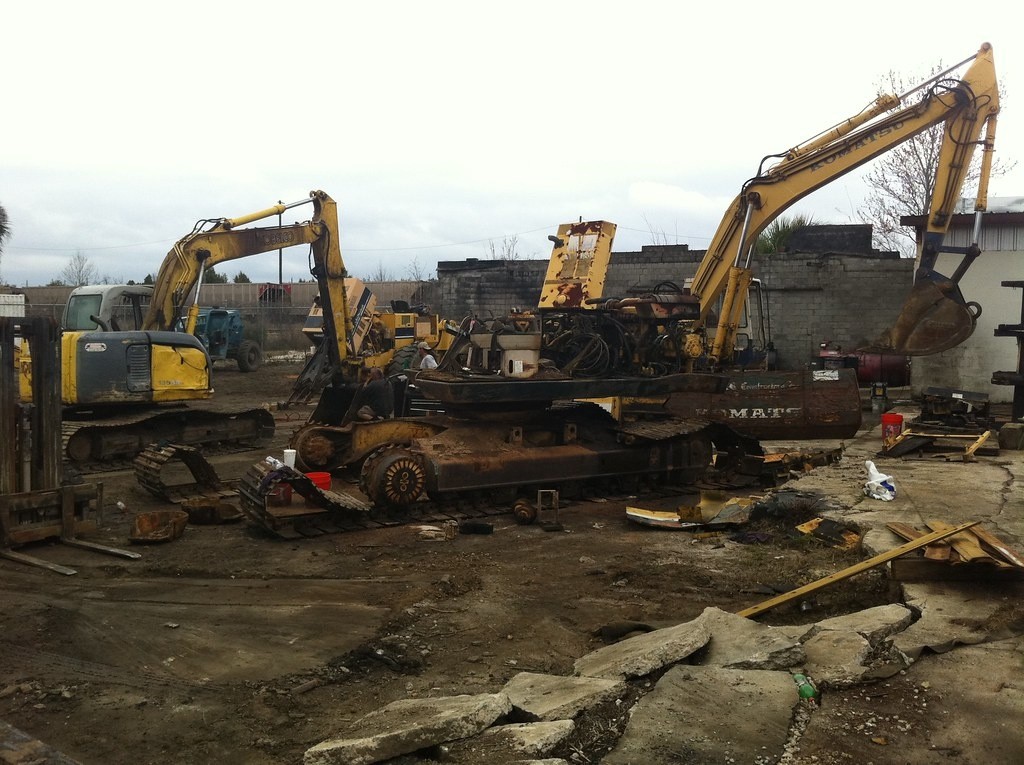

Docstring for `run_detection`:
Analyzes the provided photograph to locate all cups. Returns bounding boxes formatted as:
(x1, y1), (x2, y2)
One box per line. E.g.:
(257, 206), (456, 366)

(284, 449), (296, 469)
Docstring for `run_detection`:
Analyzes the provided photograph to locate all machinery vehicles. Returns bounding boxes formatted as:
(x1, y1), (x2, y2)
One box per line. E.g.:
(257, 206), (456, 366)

(238, 42), (998, 541)
(179, 306), (262, 374)
(14, 190), (376, 477)
(301, 278), (461, 373)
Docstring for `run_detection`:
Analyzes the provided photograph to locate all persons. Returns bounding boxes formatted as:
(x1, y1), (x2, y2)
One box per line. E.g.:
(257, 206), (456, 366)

(418, 342), (437, 371)
(357, 368), (395, 421)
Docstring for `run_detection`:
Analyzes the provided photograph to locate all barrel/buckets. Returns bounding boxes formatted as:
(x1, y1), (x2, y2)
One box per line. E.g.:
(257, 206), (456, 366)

(306, 472), (330, 491)
(881, 413), (903, 439)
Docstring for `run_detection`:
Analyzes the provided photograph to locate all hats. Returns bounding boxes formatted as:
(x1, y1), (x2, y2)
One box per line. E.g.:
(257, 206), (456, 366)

(417, 342), (431, 350)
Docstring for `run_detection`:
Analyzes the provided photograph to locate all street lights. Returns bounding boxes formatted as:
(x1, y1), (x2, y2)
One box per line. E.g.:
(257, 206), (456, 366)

(273, 200), (286, 285)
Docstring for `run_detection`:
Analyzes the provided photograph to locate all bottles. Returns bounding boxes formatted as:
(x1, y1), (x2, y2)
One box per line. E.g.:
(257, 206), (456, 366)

(794, 675), (816, 702)
(117, 502), (128, 512)
